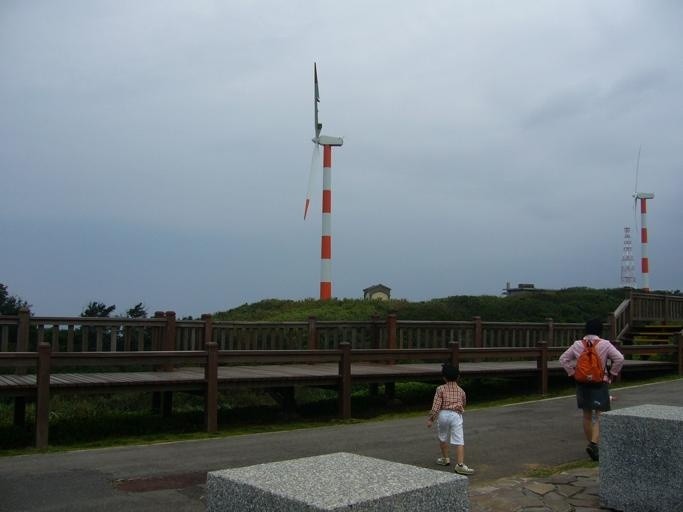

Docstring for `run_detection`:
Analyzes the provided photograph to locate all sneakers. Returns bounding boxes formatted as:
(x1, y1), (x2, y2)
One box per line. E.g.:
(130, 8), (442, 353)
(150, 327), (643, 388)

(436, 458), (450, 466)
(585, 443), (599, 461)
(454, 464), (474, 475)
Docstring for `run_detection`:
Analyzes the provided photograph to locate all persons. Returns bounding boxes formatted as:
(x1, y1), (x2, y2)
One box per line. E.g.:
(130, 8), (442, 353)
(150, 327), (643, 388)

(424, 361), (474, 476)
(555, 317), (625, 462)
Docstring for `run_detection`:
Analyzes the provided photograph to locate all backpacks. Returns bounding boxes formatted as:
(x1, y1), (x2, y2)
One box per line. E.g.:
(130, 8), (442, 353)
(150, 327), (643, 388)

(574, 339), (605, 384)
